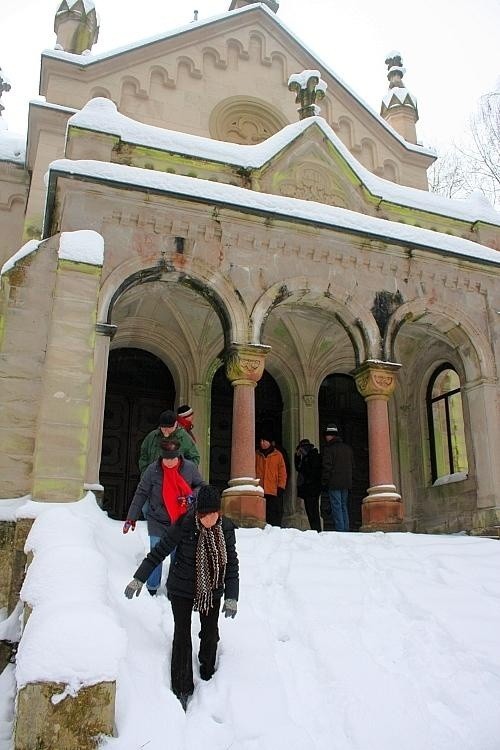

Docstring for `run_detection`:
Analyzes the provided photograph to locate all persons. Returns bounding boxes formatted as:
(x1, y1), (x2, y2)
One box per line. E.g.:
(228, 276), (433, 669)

(319, 422), (355, 531)
(138, 409), (200, 522)
(254, 431), (288, 528)
(122, 438), (206, 597)
(123, 484), (241, 712)
(174, 404), (197, 440)
(294, 438), (324, 533)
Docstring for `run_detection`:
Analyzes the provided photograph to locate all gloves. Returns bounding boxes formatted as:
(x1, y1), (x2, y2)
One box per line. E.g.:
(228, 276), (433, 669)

(177, 495), (195, 507)
(122, 520), (137, 535)
(277, 487), (284, 496)
(222, 598), (238, 620)
(123, 579), (145, 600)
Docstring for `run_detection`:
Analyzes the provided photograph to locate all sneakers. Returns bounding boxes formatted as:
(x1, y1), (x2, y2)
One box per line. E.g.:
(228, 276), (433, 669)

(177, 694), (189, 713)
(148, 589), (157, 596)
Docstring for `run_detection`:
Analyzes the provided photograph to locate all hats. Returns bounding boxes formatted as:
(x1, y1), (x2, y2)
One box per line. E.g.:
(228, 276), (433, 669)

(177, 405), (193, 419)
(324, 422), (339, 436)
(159, 409), (177, 425)
(296, 438), (314, 451)
(261, 435), (272, 443)
(197, 485), (222, 514)
